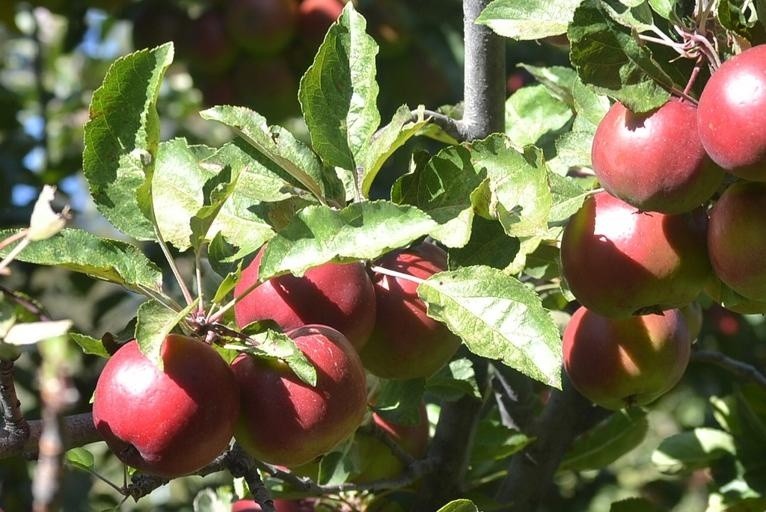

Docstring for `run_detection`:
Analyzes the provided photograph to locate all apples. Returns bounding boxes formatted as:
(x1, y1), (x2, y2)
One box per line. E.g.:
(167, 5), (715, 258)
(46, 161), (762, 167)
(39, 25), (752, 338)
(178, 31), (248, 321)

(188, 0), (345, 110)
(561, 42), (766, 413)
(93, 234), (463, 512)
